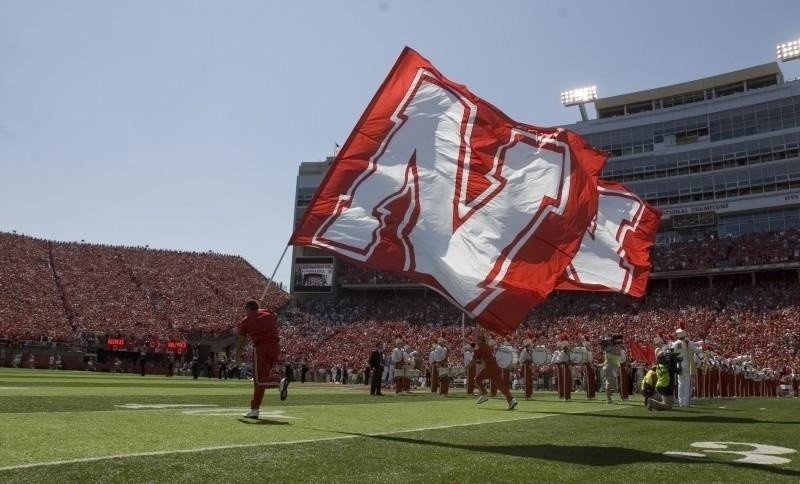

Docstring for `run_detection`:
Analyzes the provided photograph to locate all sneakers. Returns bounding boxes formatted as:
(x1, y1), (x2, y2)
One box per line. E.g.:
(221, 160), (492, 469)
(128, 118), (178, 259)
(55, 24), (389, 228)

(647, 397), (653, 410)
(279, 376), (289, 401)
(507, 398), (517, 409)
(244, 410), (258, 418)
(370, 391), (383, 395)
(476, 394), (488, 403)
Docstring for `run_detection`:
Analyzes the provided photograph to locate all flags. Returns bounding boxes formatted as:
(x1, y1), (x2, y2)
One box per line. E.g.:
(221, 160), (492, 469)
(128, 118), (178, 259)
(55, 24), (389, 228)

(289, 47), (610, 337)
(552, 177), (662, 298)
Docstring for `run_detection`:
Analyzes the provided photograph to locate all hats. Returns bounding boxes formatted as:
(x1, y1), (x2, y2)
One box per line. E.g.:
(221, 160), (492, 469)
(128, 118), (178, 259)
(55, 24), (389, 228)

(396, 338), (410, 350)
(489, 339), (510, 347)
(523, 339), (532, 345)
(582, 341), (591, 346)
(676, 328), (688, 337)
(432, 338), (446, 348)
(556, 340), (569, 349)
(653, 336), (664, 344)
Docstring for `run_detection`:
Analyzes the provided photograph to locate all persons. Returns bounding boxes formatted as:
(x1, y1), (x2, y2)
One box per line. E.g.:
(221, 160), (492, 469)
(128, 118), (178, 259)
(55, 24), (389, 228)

(230, 300), (290, 419)
(1, 229), (799, 408)
